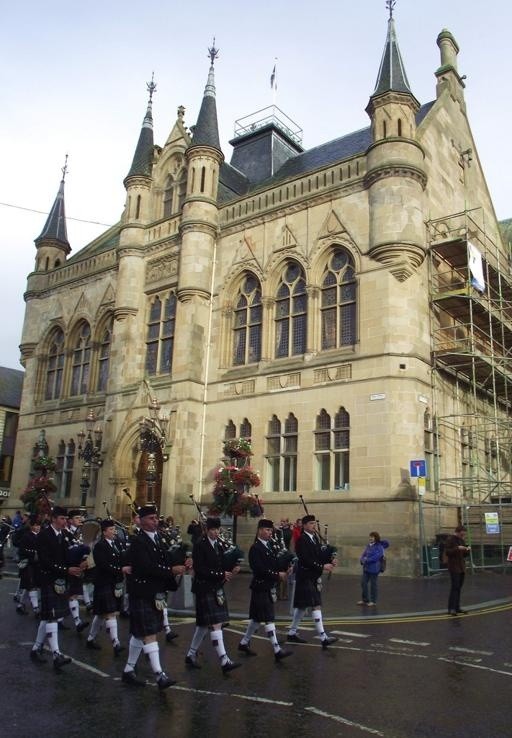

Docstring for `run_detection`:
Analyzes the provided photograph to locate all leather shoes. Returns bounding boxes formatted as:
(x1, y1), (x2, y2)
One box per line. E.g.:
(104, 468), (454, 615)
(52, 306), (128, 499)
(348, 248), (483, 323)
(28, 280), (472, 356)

(58, 604), (94, 631)
(238, 641), (256, 656)
(275, 648), (293, 661)
(222, 661), (241, 674)
(186, 654), (201, 668)
(157, 674), (175, 690)
(54, 655), (71, 668)
(87, 638), (102, 650)
(287, 634), (307, 643)
(122, 669), (147, 687)
(13, 594), (41, 618)
(322, 636), (338, 646)
(166, 630), (177, 642)
(114, 644), (124, 656)
(31, 650), (47, 663)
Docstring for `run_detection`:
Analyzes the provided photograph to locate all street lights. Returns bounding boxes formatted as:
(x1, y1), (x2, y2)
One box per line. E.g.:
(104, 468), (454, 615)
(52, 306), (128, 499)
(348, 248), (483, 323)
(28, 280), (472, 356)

(76, 406), (106, 516)
(139, 396), (171, 514)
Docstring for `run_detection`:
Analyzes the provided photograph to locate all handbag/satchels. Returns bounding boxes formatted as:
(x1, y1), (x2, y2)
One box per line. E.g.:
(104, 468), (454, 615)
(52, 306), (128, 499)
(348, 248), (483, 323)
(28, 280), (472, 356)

(381, 557), (386, 571)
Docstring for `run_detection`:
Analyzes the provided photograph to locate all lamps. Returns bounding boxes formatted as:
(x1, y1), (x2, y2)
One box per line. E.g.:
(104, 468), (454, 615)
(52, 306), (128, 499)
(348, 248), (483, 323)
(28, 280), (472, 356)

(96, 459), (104, 467)
(161, 453), (170, 462)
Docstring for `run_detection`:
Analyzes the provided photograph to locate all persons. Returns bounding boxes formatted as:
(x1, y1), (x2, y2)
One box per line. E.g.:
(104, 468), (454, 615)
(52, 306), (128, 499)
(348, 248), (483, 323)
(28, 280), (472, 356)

(444, 525), (472, 617)
(356, 531), (384, 607)
(185, 518), (241, 677)
(240, 514), (336, 662)
(121, 506), (176, 690)
(2, 506), (192, 668)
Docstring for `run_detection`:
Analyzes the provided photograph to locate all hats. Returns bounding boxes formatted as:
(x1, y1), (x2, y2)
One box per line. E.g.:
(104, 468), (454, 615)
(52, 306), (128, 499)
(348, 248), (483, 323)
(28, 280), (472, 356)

(51, 506), (67, 516)
(139, 506), (156, 517)
(207, 518), (220, 528)
(260, 519), (273, 528)
(100, 520), (114, 527)
(69, 510), (81, 515)
(302, 515), (315, 524)
